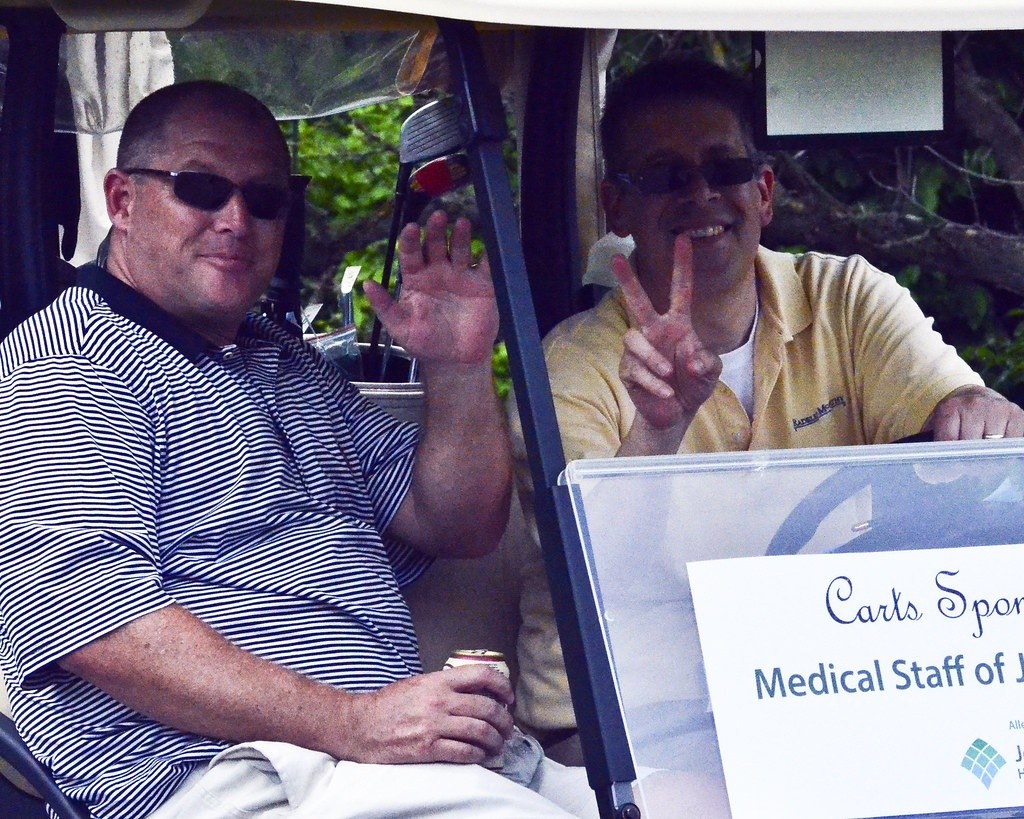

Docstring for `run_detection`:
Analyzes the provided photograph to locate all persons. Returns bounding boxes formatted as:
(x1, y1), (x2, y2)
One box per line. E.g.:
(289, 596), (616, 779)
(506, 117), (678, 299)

(0, 81), (600, 819)
(504, 56), (1024, 767)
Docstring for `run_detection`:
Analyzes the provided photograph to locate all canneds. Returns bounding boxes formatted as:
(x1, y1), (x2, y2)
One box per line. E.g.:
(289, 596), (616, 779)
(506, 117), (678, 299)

(442, 647), (510, 769)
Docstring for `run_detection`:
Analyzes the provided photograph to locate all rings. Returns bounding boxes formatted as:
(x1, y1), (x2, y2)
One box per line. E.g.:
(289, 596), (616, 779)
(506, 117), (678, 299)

(982, 434), (1007, 439)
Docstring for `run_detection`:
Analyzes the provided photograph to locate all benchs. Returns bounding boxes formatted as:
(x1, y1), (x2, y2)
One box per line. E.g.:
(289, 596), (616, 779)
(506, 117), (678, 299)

(347, 382), (542, 752)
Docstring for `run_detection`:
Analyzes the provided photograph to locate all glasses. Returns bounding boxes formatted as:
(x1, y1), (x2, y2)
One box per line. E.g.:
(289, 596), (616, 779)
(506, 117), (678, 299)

(119, 166), (311, 220)
(611, 160), (764, 192)
